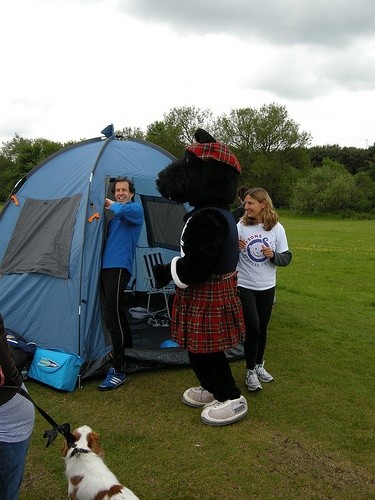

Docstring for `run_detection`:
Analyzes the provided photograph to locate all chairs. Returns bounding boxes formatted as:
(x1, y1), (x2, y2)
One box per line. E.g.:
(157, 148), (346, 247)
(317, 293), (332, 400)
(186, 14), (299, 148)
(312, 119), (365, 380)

(141, 253), (176, 320)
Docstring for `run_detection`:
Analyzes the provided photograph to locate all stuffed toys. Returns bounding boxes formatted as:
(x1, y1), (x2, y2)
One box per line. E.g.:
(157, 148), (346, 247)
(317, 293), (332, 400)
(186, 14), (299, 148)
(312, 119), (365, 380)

(153, 128), (249, 424)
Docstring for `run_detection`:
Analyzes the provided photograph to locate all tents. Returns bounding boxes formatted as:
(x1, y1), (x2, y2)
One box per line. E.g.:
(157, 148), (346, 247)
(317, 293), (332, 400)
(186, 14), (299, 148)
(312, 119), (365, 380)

(1, 122), (244, 392)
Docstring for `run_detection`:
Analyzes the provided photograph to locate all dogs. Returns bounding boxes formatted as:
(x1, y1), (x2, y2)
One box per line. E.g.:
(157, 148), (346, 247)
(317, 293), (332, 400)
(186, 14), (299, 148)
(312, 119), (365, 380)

(59, 424), (140, 500)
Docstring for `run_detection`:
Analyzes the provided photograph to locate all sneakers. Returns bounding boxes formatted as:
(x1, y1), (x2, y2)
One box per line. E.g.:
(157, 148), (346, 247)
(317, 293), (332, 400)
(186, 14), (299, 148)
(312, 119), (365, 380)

(98, 368), (126, 390)
(256, 360), (275, 383)
(246, 365), (263, 391)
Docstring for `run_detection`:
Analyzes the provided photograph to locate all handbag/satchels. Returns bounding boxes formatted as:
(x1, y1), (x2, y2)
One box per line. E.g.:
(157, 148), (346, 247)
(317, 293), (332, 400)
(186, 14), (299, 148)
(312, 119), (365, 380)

(28, 348), (83, 391)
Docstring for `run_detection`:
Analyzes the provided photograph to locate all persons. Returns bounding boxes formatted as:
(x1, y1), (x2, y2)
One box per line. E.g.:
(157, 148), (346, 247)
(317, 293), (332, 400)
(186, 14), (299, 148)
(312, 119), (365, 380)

(0, 315), (36, 500)
(98, 177), (144, 393)
(232, 186), (293, 392)
